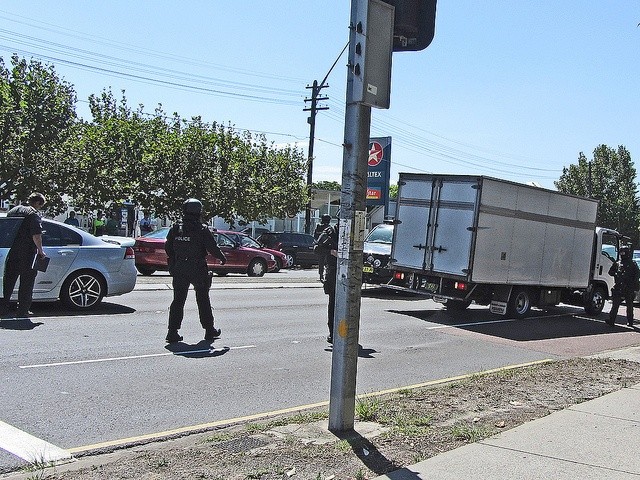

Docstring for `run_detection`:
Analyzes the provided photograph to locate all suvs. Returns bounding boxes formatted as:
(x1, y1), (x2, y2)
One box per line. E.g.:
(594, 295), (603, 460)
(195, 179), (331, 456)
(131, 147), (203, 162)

(260, 232), (321, 269)
(361, 223), (422, 297)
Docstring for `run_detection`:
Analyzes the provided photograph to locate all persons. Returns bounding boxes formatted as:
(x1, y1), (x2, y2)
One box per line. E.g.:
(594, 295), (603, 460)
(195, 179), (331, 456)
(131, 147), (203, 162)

(163, 197), (223, 341)
(93, 210), (106, 234)
(314, 206), (341, 343)
(1, 190), (45, 317)
(63, 208), (78, 227)
(313, 214), (332, 280)
(139, 209), (152, 234)
(105, 208), (120, 234)
(603, 245), (639, 327)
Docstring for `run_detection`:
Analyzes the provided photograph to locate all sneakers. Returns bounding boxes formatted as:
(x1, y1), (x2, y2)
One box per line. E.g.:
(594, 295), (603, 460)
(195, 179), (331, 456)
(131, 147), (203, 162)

(627, 321), (633, 325)
(605, 319), (614, 327)
(205, 328), (221, 339)
(166, 331), (183, 341)
(16, 306), (34, 317)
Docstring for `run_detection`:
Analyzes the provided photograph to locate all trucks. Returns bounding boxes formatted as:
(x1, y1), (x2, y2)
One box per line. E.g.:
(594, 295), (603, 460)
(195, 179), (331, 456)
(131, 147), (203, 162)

(379, 172), (623, 320)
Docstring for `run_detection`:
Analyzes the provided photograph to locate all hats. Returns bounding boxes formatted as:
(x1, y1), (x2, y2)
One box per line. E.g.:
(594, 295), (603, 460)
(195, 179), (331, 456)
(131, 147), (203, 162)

(181, 199), (202, 213)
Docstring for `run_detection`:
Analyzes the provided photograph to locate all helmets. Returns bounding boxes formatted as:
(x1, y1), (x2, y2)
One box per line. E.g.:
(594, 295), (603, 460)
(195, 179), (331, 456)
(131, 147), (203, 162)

(322, 214), (331, 223)
(620, 247), (629, 259)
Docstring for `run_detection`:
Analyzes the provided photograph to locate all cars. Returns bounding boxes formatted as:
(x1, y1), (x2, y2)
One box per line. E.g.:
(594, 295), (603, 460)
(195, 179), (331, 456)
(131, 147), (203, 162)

(131, 226), (276, 277)
(621, 258), (640, 304)
(1, 212), (139, 311)
(219, 230), (287, 273)
(632, 250), (640, 258)
(237, 227), (272, 238)
